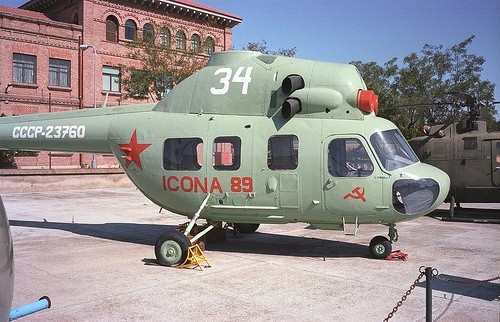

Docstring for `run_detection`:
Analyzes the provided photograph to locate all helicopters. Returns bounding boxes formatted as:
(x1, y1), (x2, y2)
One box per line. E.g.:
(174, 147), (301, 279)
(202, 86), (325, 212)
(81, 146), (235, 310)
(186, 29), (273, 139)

(3, 49), (451, 265)
(405, 88), (500, 224)
